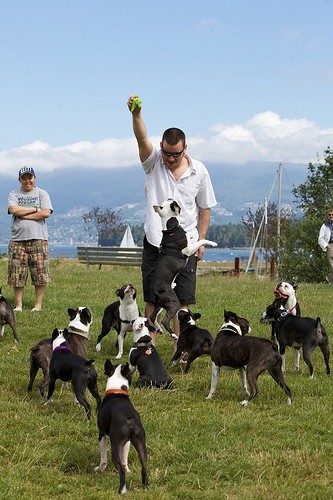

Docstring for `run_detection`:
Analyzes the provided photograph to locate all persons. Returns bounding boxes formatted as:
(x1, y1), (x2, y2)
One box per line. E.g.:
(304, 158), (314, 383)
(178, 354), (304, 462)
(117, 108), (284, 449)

(127, 95), (217, 363)
(318, 208), (333, 284)
(7, 166), (54, 312)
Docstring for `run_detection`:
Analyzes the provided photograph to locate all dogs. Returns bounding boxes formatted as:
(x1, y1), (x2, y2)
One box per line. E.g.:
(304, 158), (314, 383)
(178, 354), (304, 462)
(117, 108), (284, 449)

(261, 297), (331, 379)
(42, 328), (103, 421)
(95, 282), (141, 359)
(129, 317), (174, 390)
(0, 288), (20, 343)
(204, 310), (294, 406)
(95, 358), (149, 493)
(169, 308), (214, 373)
(28, 307), (94, 398)
(146, 199), (218, 341)
(260, 281), (301, 325)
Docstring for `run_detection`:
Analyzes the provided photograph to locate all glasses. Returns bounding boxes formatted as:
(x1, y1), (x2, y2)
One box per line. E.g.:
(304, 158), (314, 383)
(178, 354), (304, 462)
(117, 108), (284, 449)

(162, 144), (185, 157)
(328, 214), (333, 216)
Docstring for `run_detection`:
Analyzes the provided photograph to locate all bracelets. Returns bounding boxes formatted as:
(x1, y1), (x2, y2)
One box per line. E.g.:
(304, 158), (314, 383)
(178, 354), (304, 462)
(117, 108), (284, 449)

(35, 207), (38, 212)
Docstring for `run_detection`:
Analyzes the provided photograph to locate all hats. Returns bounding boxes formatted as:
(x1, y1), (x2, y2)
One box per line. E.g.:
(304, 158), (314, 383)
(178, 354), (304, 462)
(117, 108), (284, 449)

(18, 167), (35, 178)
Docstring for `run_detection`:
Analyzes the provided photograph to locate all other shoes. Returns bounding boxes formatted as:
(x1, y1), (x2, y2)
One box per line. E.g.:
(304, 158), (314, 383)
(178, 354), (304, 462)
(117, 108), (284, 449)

(31, 308), (40, 312)
(13, 309), (21, 312)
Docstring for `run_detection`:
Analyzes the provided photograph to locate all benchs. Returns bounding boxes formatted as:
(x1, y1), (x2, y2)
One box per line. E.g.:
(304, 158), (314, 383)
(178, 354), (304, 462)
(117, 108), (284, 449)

(76, 247), (143, 271)
